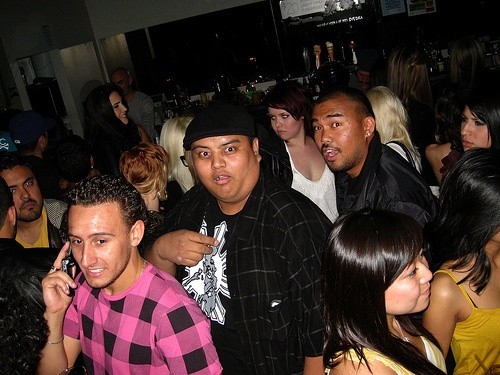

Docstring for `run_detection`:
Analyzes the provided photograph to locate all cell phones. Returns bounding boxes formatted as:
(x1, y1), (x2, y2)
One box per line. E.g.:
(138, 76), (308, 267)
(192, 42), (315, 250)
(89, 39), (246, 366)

(61, 244), (77, 297)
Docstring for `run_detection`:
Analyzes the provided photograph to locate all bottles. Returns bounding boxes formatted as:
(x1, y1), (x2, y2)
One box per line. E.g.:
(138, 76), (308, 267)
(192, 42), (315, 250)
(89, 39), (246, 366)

(246, 81), (254, 104)
(436, 50), (445, 74)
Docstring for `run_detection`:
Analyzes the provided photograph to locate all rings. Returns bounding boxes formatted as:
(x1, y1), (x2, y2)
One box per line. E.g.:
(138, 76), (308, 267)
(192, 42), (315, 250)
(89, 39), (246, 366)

(50, 264), (60, 271)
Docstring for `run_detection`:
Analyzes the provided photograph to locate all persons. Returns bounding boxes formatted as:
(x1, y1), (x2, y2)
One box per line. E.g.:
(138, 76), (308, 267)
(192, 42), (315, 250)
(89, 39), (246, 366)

(0, 36), (500, 375)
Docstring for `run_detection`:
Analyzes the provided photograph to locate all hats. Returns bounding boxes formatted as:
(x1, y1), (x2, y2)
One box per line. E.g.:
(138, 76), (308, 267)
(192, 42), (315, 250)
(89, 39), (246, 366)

(183, 102), (257, 152)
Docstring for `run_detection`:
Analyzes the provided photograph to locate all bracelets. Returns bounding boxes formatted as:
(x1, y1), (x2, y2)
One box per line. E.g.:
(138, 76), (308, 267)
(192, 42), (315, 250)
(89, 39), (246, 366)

(46, 337), (65, 344)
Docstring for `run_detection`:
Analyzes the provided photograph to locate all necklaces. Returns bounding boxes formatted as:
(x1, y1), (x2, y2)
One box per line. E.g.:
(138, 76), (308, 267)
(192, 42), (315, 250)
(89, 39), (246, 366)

(141, 258), (146, 274)
